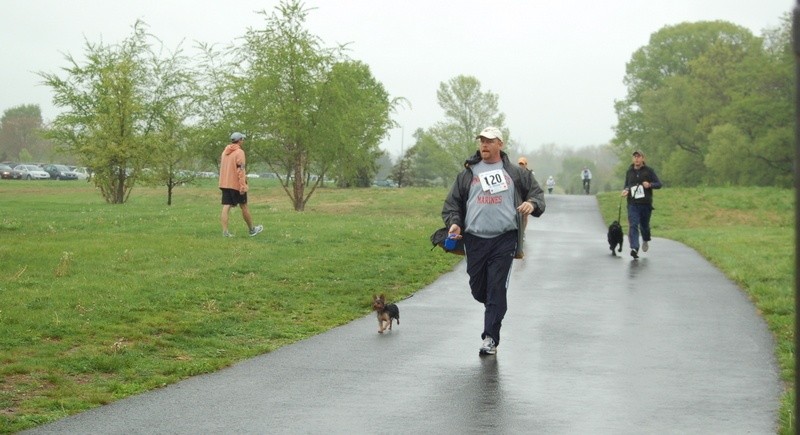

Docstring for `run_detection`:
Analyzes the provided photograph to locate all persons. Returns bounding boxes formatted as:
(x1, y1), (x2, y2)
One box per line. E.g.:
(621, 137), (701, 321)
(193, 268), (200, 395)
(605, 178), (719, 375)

(518, 157), (592, 242)
(218, 132), (263, 240)
(440, 127), (546, 356)
(621, 150), (663, 260)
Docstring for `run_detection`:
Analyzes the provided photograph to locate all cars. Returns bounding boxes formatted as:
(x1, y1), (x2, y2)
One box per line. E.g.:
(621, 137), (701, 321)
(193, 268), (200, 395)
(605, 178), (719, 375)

(44, 164), (78, 181)
(0, 161), (16, 180)
(67, 165), (132, 180)
(140, 167), (219, 179)
(14, 162), (52, 180)
(310, 175), (336, 182)
(246, 172), (295, 181)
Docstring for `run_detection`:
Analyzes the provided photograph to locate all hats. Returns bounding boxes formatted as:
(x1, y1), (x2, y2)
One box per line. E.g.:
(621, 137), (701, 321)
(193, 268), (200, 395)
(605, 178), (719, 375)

(231, 132), (246, 142)
(518, 158), (527, 165)
(632, 151), (644, 157)
(476, 127), (503, 143)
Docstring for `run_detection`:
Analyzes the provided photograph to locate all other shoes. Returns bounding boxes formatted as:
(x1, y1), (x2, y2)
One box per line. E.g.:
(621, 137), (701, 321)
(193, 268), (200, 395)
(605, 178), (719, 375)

(630, 249), (639, 258)
(249, 225), (263, 237)
(642, 241), (648, 252)
(480, 335), (497, 355)
(223, 231), (231, 237)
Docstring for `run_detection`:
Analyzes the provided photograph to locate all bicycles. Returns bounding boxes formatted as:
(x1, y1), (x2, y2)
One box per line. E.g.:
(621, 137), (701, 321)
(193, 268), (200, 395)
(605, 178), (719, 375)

(582, 179), (592, 195)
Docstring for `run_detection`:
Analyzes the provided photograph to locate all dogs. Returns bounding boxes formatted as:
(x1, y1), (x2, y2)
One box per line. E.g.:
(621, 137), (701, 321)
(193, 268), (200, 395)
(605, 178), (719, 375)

(372, 293), (399, 333)
(608, 220), (623, 256)
